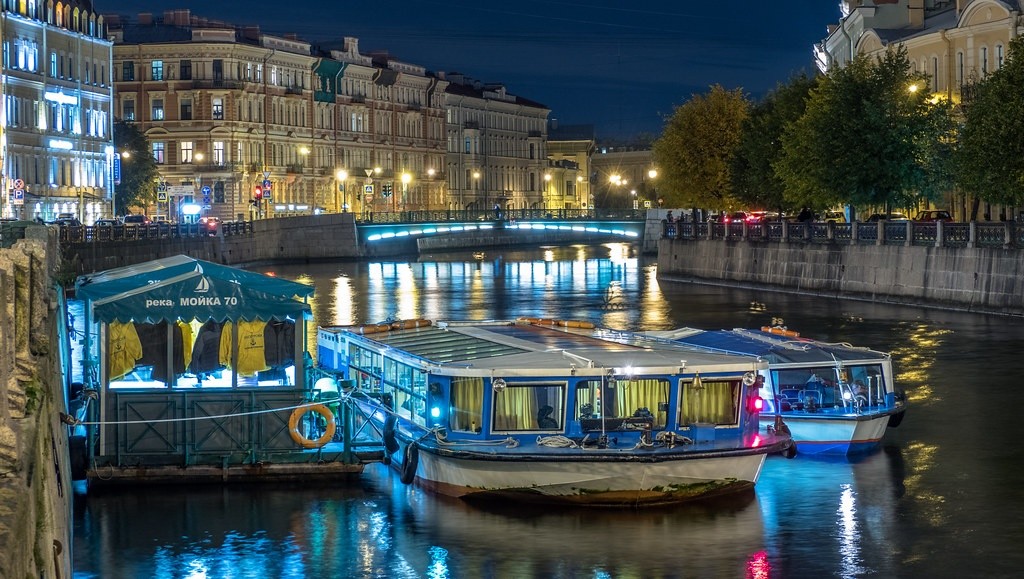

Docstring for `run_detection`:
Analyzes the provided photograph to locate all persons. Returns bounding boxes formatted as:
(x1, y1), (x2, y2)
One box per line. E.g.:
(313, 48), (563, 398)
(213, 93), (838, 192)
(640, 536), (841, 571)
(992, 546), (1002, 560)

(794, 205), (814, 222)
(666, 210), (674, 222)
(851, 380), (868, 397)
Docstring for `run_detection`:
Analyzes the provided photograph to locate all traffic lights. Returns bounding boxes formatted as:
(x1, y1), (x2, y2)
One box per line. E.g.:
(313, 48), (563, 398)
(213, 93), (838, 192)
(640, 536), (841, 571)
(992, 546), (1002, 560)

(256, 186), (262, 199)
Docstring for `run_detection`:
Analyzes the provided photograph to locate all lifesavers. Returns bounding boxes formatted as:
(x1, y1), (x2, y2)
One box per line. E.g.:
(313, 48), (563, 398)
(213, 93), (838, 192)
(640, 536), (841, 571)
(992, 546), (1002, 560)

(347, 318), (432, 334)
(760, 325), (801, 337)
(287, 403), (337, 448)
(516, 317), (596, 329)
(888, 387), (906, 428)
(400, 443), (419, 485)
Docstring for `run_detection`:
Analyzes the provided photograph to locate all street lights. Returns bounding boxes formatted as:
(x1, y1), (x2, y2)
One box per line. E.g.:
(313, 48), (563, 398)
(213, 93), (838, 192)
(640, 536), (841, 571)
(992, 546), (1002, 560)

(337, 170), (346, 212)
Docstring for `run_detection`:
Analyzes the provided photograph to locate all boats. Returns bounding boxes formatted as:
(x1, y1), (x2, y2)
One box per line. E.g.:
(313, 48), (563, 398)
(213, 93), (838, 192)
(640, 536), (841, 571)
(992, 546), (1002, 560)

(641, 325), (909, 460)
(75, 253), (373, 484)
(316, 312), (792, 506)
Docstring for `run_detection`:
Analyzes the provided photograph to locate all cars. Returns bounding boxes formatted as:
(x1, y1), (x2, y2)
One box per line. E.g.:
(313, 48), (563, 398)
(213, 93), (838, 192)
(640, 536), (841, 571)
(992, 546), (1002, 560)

(704, 210), (848, 234)
(157, 220), (177, 234)
(53, 217), (83, 237)
(92, 219), (123, 237)
(861, 212), (909, 235)
(912, 210), (956, 236)
(196, 216), (221, 233)
(123, 215), (155, 235)
